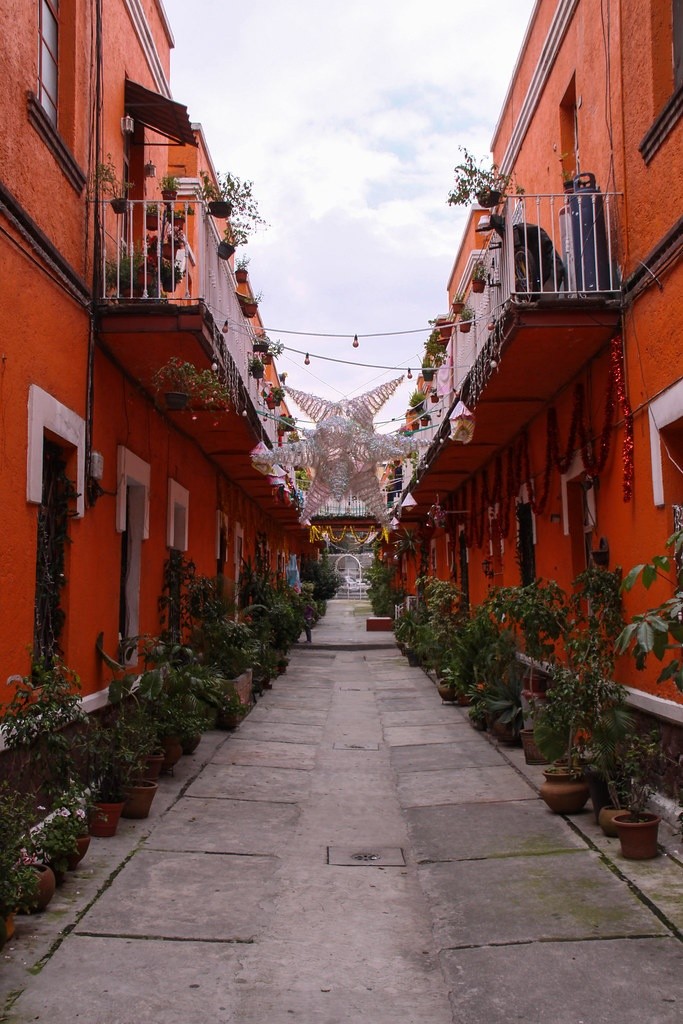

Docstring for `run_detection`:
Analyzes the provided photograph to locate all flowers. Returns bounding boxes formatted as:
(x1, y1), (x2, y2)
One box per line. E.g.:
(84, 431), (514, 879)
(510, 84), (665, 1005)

(153, 356), (230, 427)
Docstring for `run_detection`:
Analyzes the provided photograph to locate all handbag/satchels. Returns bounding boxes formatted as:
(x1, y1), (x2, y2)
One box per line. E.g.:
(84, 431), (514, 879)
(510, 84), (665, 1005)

(512, 222), (566, 301)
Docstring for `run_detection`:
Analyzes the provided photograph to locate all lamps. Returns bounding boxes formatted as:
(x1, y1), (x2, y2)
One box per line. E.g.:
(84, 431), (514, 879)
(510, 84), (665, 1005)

(145, 160), (156, 177)
(121, 112), (134, 136)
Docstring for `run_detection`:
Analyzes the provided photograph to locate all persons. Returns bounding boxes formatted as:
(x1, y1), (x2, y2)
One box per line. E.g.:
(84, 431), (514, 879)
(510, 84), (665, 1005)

(387, 476), (396, 508)
(302, 597), (316, 643)
(503, 223), (566, 302)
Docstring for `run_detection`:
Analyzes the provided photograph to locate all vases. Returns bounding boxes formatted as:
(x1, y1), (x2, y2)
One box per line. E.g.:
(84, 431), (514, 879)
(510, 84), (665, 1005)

(163, 392), (190, 410)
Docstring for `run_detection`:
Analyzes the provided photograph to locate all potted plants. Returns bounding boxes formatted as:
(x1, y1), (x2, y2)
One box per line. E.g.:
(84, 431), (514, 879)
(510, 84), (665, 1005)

(0, 550), (318, 945)
(91, 162), (309, 490)
(390, 146), (579, 478)
(390, 566), (664, 859)
